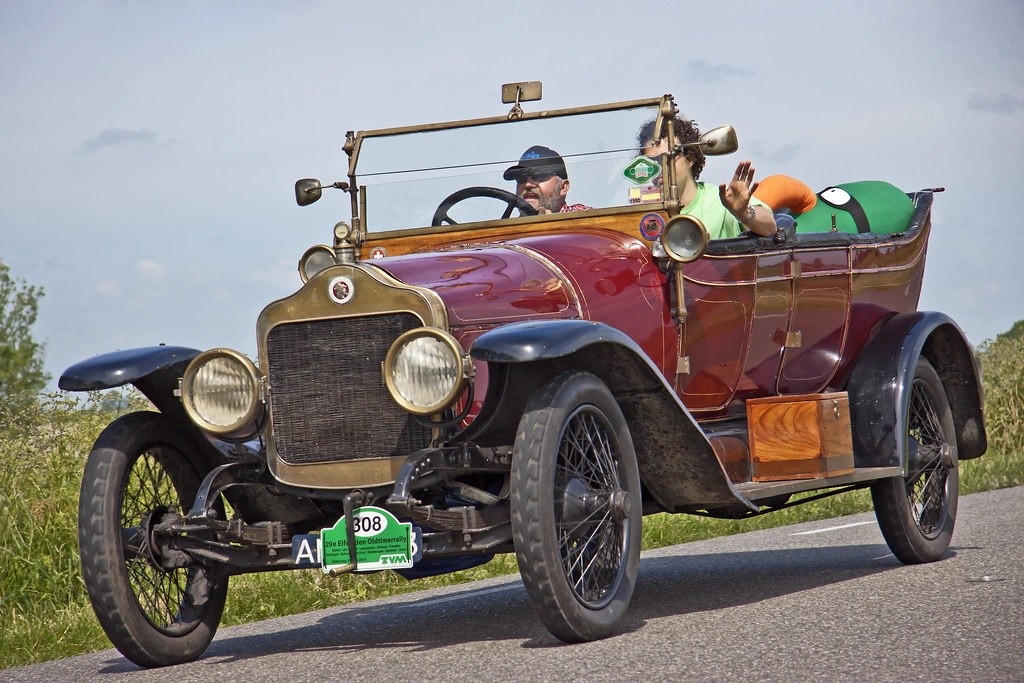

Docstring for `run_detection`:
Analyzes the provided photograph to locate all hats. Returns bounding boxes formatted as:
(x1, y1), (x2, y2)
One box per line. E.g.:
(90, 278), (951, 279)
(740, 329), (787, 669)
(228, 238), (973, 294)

(503, 145), (567, 180)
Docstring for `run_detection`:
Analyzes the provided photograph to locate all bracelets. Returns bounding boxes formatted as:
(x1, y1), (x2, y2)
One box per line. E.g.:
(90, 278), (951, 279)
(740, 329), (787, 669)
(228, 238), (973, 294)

(741, 206), (755, 224)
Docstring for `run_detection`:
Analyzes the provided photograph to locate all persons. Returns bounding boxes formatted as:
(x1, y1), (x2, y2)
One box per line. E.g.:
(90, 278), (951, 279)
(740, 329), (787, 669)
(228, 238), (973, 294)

(503, 146), (593, 216)
(637, 118), (777, 240)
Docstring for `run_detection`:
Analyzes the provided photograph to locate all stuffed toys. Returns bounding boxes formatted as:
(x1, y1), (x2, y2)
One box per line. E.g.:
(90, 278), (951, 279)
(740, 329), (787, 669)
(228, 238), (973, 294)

(749, 174), (916, 234)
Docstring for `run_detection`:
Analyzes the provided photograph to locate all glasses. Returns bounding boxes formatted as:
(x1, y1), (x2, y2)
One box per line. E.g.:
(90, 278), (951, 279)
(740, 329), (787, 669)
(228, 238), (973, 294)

(513, 171), (557, 184)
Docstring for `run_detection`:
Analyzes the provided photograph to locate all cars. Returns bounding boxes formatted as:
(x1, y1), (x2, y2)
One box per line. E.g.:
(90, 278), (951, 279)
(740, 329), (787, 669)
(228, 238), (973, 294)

(58, 79), (988, 669)
